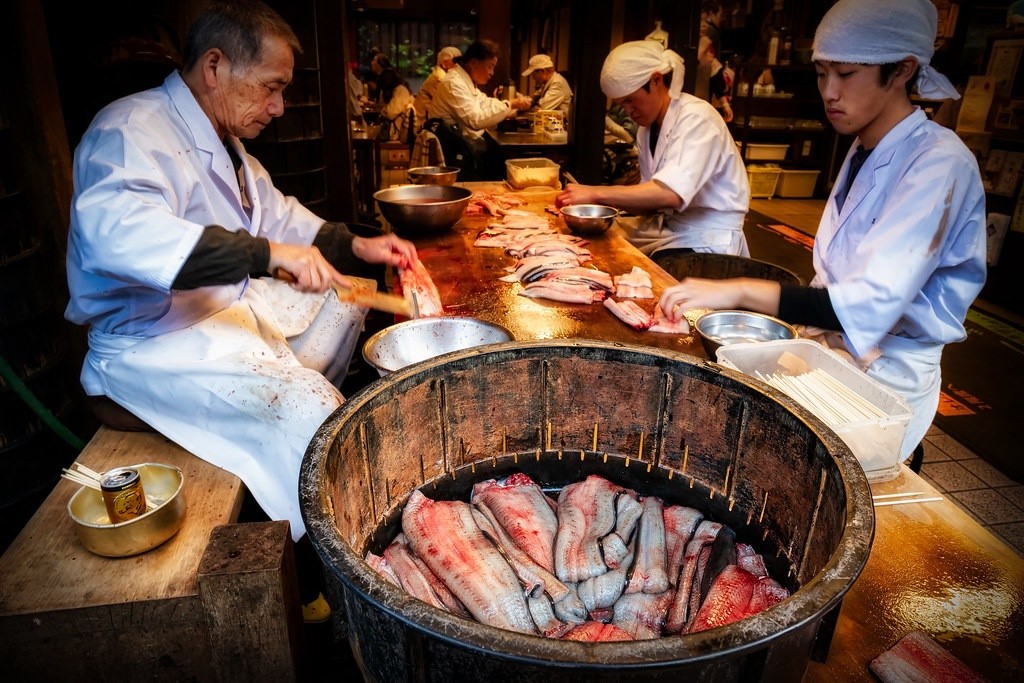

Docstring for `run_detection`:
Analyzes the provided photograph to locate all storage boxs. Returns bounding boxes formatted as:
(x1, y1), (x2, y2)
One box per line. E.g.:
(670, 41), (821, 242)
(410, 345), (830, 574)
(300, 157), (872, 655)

(745, 169), (781, 199)
(777, 170), (821, 198)
(734, 141), (789, 161)
(504, 158), (561, 188)
(715, 339), (914, 479)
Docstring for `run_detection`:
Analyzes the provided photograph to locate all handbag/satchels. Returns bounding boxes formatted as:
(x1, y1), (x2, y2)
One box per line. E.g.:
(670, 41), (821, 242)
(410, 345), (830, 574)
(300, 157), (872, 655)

(379, 116), (390, 142)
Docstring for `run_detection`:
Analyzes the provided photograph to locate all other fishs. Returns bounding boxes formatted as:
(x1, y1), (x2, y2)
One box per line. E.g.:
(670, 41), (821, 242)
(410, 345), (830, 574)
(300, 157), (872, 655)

(364, 474), (790, 643)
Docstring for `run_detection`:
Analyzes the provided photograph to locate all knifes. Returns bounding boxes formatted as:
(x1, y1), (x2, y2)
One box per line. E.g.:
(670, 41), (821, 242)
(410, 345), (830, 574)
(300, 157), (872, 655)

(272, 269), (412, 316)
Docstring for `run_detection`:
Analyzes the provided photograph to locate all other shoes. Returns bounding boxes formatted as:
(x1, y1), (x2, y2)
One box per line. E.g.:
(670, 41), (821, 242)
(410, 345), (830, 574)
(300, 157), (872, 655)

(288, 609), (366, 683)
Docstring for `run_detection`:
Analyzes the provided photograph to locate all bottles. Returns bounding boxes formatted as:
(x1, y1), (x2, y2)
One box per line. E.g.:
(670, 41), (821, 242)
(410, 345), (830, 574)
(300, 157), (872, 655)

(503, 78), (516, 101)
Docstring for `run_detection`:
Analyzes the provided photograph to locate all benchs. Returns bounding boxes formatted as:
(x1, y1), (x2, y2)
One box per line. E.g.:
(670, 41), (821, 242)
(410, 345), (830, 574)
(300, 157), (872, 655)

(0, 423), (303, 683)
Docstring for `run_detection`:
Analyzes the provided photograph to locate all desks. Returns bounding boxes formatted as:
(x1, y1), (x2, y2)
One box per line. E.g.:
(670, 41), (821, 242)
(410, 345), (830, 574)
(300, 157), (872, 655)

(351, 123), (383, 216)
(388, 176), (1024, 683)
(485, 126), (633, 179)
(361, 104), (386, 122)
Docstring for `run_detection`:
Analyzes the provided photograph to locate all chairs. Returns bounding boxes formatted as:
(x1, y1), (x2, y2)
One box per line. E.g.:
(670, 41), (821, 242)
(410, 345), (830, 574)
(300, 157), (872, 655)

(404, 104), (474, 182)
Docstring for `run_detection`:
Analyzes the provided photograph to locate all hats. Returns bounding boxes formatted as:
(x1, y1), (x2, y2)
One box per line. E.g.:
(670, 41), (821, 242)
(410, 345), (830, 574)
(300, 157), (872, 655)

(521, 54), (554, 77)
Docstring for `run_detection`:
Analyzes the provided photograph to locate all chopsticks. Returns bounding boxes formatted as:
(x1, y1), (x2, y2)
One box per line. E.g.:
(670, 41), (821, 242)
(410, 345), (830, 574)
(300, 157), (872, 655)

(872, 491), (943, 504)
(752, 368), (891, 428)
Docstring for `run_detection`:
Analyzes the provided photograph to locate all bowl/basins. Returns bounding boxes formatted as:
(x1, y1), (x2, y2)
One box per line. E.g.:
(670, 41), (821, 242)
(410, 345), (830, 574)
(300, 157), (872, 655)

(67, 463), (186, 558)
(298, 340), (874, 683)
(559, 204), (619, 235)
(658, 254), (807, 287)
(362, 316), (515, 378)
(373, 184), (474, 232)
(407, 166), (461, 186)
(694, 309), (798, 363)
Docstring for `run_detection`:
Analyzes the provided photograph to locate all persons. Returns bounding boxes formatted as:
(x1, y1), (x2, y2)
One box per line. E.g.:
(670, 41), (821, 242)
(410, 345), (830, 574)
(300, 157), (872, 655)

(66, 0), (417, 623)
(362, 38), (572, 183)
(605, 0), (750, 185)
(659, 0), (987, 464)
(554, 41), (751, 259)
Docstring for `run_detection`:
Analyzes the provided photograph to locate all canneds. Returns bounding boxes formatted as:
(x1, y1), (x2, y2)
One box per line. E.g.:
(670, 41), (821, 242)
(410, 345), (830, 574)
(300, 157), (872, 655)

(100, 466), (148, 525)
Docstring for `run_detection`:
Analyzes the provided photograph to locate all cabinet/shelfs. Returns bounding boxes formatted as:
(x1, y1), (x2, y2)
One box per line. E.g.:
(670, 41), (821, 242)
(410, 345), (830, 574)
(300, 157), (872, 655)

(729, 54), (832, 200)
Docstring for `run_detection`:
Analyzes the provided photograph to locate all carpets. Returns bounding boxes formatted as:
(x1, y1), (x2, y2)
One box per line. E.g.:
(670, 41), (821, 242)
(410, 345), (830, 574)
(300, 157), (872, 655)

(744, 210), (1023, 488)
